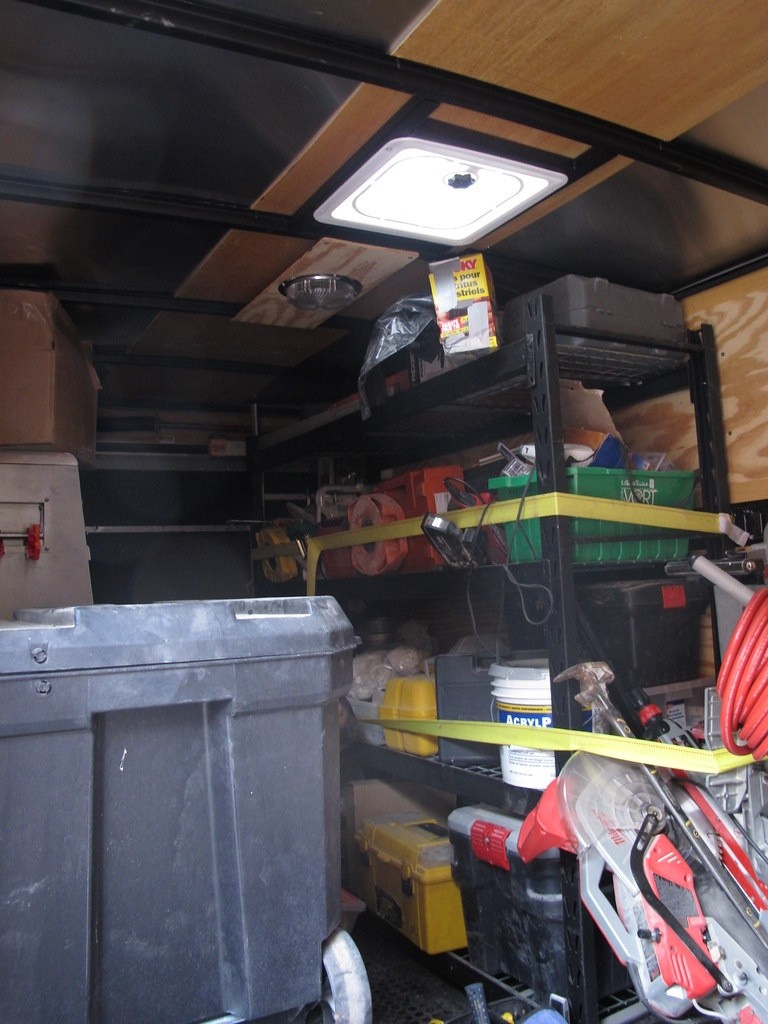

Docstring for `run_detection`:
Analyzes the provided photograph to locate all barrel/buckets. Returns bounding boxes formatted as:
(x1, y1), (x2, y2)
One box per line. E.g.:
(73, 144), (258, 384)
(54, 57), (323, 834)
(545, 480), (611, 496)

(489, 658), (556, 790)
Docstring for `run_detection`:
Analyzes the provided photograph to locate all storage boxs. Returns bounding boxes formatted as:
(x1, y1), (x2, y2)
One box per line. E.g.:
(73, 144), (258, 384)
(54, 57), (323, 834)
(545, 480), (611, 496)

(0, 251), (701, 1024)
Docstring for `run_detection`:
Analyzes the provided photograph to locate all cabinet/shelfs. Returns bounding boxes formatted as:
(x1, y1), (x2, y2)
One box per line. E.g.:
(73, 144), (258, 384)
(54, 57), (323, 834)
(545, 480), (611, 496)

(248, 295), (730, 1022)
